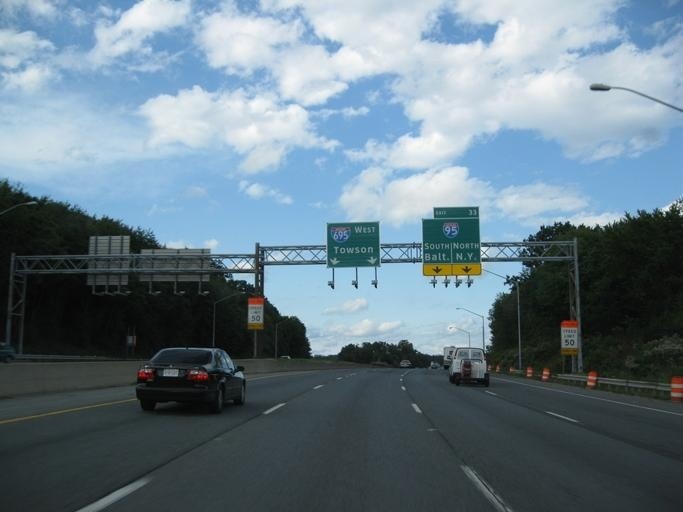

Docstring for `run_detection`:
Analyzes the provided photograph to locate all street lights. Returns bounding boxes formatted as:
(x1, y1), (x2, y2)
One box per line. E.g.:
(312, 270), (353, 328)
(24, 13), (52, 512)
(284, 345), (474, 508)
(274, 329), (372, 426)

(590, 82), (683, 112)
(449, 308), (485, 351)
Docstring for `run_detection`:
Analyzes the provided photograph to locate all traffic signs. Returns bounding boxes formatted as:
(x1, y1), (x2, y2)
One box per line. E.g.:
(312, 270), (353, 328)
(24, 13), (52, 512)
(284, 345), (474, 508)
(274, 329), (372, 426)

(327, 222), (380, 267)
(423, 218), (481, 275)
(247, 296), (265, 330)
(560, 320), (578, 354)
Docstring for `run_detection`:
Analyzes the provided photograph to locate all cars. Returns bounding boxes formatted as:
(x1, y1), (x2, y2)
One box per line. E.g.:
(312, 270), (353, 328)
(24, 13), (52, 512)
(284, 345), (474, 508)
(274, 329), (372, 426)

(400, 360), (412, 368)
(135, 347), (245, 413)
(0, 342), (16, 363)
(431, 361), (440, 369)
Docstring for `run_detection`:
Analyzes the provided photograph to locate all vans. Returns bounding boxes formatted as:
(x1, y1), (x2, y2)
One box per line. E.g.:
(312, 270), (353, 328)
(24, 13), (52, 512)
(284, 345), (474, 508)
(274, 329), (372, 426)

(443, 346), (489, 387)
